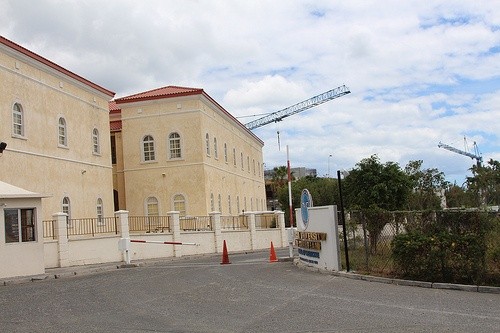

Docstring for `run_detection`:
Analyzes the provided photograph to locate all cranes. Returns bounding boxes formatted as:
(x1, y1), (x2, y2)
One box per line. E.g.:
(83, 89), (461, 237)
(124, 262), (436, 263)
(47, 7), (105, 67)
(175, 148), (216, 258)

(233, 84), (351, 151)
(438, 135), (483, 166)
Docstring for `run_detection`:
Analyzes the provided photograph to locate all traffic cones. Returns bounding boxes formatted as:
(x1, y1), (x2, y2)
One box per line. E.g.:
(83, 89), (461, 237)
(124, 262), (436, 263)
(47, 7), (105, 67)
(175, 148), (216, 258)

(219, 240), (231, 264)
(268, 241), (279, 263)
